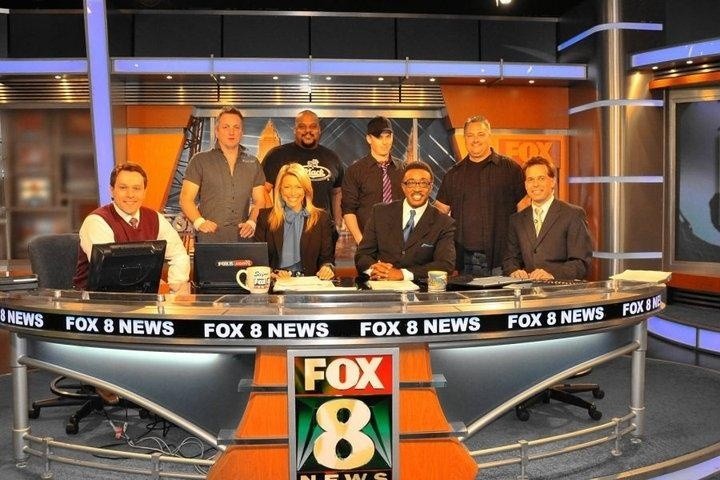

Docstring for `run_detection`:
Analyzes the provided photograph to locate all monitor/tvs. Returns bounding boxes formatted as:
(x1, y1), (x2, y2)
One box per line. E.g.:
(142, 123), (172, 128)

(674, 100), (720, 263)
(86, 240), (167, 294)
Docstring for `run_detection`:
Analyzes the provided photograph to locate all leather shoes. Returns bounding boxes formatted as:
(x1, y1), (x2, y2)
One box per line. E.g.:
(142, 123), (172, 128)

(94, 387), (119, 405)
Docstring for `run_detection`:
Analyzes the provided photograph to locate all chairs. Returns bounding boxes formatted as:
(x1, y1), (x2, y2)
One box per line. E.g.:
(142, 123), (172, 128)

(26, 231), (165, 438)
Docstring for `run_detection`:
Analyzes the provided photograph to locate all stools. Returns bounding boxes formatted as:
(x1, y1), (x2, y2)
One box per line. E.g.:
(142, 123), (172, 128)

(512, 363), (606, 425)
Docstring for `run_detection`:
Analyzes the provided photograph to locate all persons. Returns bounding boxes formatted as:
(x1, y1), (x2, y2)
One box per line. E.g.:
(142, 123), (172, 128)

(255, 162), (338, 280)
(179, 106), (267, 243)
(72, 159), (193, 405)
(501, 155), (596, 281)
(260, 108), (343, 237)
(434, 115), (524, 273)
(343, 115), (408, 248)
(352, 160), (457, 281)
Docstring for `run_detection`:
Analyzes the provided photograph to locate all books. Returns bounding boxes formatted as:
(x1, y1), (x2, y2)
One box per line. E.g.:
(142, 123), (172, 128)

(366, 278), (422, 292)
(273, 275), (360, 292)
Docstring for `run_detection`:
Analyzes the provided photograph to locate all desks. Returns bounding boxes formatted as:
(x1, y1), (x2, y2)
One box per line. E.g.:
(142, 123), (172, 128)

(1, 273), (672, 480)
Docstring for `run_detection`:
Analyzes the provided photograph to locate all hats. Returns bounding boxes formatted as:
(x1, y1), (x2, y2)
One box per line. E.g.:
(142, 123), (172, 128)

(367, 116), (394, 137)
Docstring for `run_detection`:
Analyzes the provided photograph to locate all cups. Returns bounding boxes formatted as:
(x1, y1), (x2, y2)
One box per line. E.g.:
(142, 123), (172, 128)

(236, 266), (271, 295)
(428, 271), (449, 294)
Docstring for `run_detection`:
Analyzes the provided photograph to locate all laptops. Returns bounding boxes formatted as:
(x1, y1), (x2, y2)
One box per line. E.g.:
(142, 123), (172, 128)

(194, 243), (270, 290)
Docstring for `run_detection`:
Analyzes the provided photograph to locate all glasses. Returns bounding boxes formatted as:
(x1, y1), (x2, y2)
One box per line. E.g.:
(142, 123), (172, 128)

(402, 179), (432, 187)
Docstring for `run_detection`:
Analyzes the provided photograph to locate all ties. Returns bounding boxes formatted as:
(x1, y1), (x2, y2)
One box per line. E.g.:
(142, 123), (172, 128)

(533, 207), (543, 237)
(130, 218), (138, 229)
(403, 210), (416, 249)
(377, 161), (393, 204)
